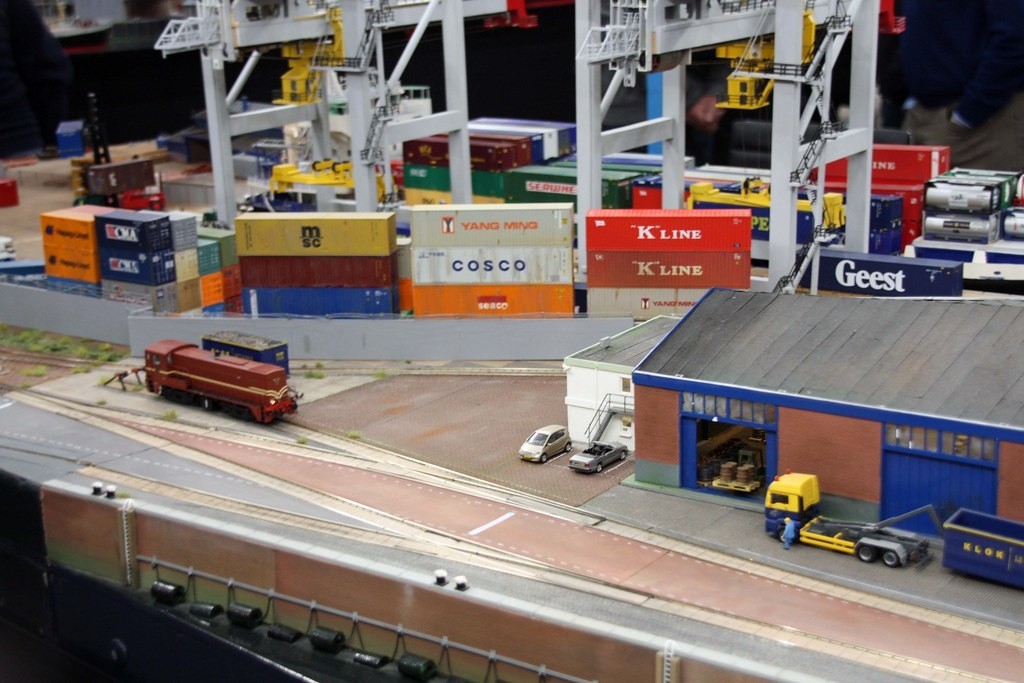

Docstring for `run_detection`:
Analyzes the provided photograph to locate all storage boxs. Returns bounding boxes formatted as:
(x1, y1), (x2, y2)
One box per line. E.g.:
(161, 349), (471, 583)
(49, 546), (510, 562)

(0, 117), (1024, 322)
(201, 332), (289, 381)
(942, 507), (1024, 592)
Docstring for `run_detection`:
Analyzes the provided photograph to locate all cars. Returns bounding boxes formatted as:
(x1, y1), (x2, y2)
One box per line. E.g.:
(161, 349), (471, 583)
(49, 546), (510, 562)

(568, 441), (628, 474)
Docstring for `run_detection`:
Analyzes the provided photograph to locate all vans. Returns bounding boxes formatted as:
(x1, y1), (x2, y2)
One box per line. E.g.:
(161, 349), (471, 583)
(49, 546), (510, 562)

(518, 424), (570, 463)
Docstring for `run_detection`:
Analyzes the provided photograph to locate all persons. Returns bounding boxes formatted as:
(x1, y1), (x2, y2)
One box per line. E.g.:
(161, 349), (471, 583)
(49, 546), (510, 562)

(897, 1), (1024, 170)
(780, 518), (795, 550)
(602, 0), (746, 167)
(0, 0), (91, 159)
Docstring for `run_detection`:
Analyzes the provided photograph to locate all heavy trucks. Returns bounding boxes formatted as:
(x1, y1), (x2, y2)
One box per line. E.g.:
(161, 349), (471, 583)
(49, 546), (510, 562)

(144, 338), (292, 424)
(763, 470), (937, 569)
